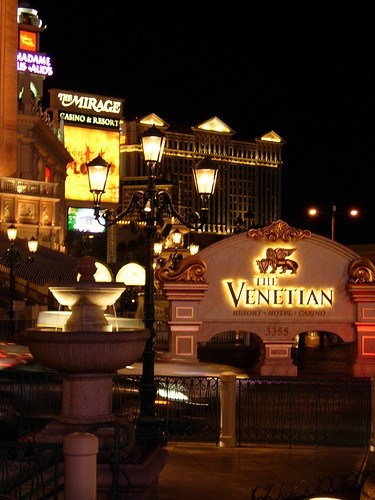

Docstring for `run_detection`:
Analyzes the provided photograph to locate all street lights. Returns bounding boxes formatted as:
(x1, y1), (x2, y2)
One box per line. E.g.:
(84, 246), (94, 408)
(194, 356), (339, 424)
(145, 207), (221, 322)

(0, 224), (38, 342)
(154, 229), (200, 271)
(308, 204), (358, 240)
(87, 124), (221, 448)
